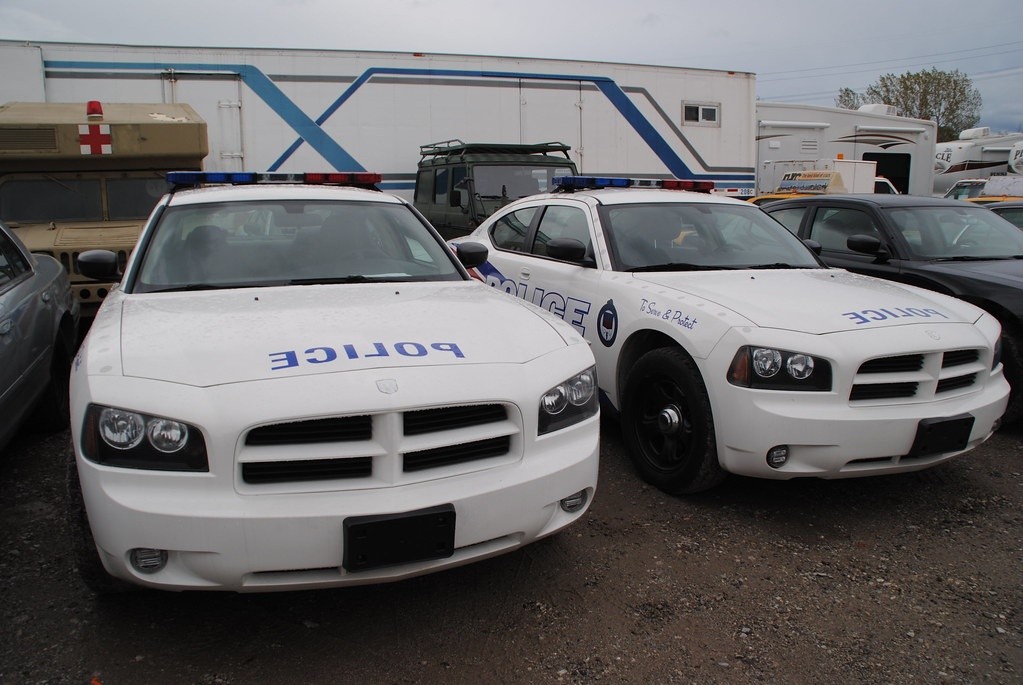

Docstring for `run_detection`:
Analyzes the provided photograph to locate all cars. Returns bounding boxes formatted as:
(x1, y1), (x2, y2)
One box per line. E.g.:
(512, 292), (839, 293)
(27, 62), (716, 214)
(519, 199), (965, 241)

(0, 220), (82, 458)
(745, 171), (1023, 426)
(440, 175), (1013, 494)
(67, 172), (603, 591)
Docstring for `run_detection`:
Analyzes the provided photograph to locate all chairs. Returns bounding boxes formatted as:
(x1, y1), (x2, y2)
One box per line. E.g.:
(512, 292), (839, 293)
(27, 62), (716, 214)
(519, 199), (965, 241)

(648, 211), (698, 264)
(305, 213), (391, 273)
(180, 226), (249, 277)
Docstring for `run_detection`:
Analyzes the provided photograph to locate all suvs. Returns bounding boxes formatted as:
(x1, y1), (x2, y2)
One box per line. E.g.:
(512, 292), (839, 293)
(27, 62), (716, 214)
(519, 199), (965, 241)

(412, 140), (582, 241)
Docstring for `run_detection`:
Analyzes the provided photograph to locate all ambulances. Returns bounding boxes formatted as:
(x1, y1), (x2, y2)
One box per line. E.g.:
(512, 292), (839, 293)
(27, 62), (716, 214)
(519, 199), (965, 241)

(0, 98), (208, 318)
